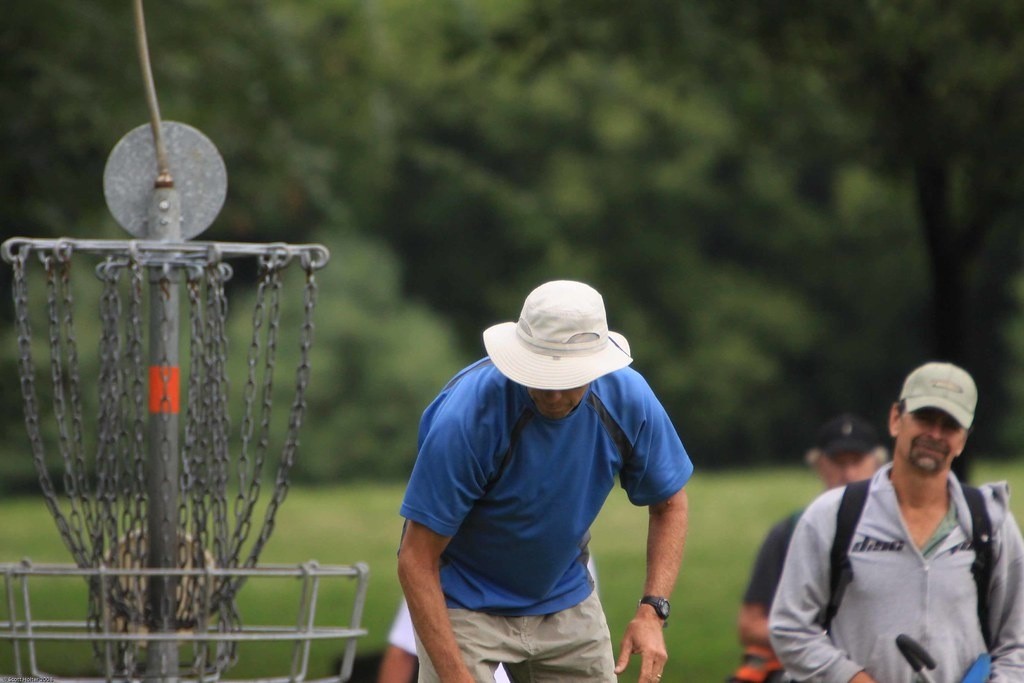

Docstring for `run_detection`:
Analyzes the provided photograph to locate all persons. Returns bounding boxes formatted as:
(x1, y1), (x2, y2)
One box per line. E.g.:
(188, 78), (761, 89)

(768, 361), (1024, 683)
(723, 413), (887, 683)
(375, 279), (693, 683)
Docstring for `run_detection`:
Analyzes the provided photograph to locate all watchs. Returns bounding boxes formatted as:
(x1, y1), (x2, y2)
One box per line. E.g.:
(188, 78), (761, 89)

(636, 596), (670, 619)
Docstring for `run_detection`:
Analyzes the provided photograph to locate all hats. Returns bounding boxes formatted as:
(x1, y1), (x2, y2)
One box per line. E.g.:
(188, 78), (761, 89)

(900, 361), (979, 429)
(481, 279), (633, 391)
(816, 416), (881, 456)
